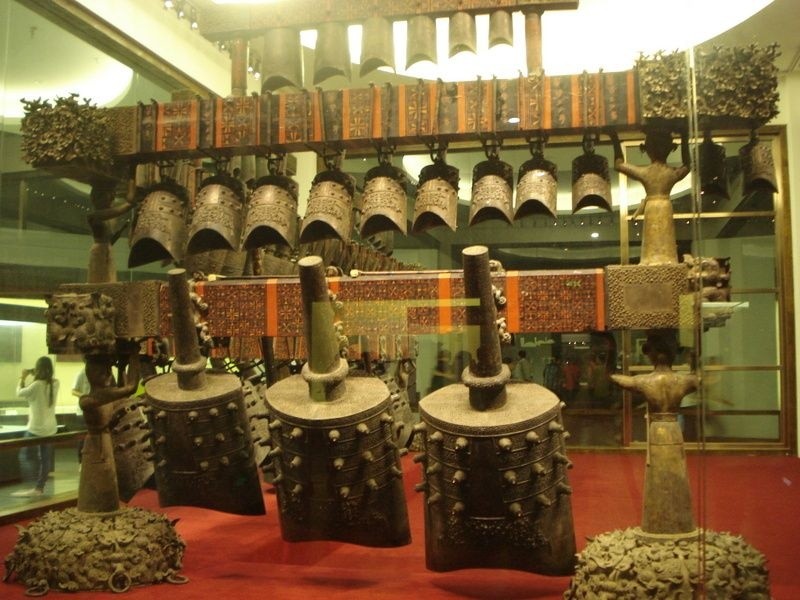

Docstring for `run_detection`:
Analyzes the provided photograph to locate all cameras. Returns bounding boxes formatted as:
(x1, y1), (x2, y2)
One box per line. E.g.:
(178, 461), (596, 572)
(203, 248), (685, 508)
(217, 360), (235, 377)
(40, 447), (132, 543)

(28, 369), (33, 373)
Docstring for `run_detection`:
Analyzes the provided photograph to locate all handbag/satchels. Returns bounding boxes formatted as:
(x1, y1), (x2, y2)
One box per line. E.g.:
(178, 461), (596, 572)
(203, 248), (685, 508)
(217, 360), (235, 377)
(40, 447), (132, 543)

(573, 383), (581, 391)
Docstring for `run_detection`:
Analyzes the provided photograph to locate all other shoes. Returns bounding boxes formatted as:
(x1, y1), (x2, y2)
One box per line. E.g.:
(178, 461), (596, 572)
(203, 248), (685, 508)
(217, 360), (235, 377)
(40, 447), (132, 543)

(11, 488), (43, 496)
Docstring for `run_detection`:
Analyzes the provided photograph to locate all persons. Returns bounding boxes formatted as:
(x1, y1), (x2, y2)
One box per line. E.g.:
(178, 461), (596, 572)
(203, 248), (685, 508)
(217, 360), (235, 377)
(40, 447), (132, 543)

(504, 359), (515, 380)
(610, 334), (698, 535)
(11, 356), (59, 497)
(78, 345), (141, 514)
(609, 127), (691, 264)
(85, 163), (140, 281)
(514, 350), (532, 383)
(73, 370), (91, 472)
(544, 353), (612, 407)
